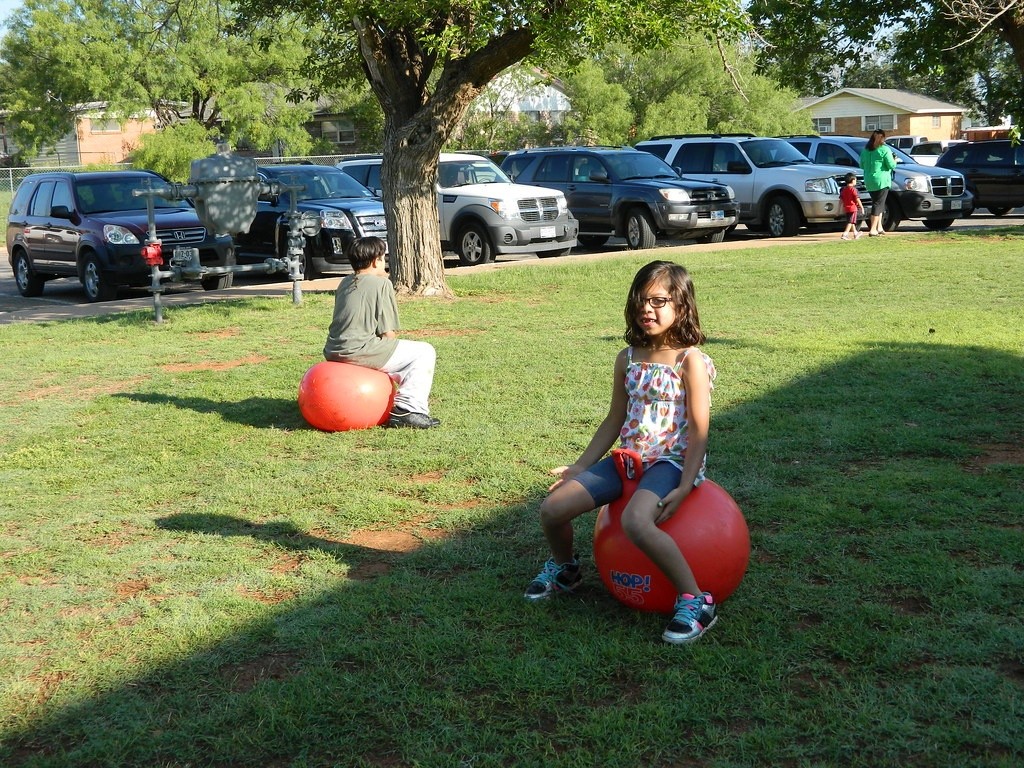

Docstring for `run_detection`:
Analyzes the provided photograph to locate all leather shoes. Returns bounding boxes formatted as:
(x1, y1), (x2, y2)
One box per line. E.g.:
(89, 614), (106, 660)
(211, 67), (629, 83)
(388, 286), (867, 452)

(389, 406), (440, 429)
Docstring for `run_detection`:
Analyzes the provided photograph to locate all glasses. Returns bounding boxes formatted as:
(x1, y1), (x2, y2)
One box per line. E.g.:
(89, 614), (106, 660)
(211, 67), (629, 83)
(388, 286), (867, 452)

(634, 296), (672, 308)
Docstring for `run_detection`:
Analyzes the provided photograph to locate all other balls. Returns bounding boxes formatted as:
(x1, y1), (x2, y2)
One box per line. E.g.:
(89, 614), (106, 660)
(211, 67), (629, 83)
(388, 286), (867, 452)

(594, 477), (751, 613)
(298, 361), (396, 432)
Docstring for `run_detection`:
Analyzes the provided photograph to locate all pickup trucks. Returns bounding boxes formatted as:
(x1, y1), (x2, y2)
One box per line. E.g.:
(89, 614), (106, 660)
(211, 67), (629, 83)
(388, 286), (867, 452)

(908, 139), (968, 166)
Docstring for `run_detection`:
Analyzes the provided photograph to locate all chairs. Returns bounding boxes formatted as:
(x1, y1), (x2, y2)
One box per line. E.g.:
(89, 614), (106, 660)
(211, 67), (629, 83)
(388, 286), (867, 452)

(305, 180), (327, 199)
(577, 164), (594, 181)
(454, 171), (470, 186)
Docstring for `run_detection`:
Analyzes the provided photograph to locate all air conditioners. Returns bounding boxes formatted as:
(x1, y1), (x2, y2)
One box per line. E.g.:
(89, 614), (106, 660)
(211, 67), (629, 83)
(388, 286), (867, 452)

(818, 126), (828, 133)
(867, 123), (876, 131)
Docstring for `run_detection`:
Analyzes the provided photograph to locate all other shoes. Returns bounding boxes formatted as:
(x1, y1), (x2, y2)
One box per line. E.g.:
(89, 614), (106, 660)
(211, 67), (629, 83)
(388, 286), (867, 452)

(854, 231), (863, 239)
(841, 234), (852, 240)
(868, 232), (881, 237)
(878, 229), (886, 235)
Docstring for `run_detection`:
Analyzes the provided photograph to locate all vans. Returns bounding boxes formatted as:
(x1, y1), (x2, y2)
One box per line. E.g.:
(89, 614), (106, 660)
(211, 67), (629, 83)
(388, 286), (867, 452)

(885, 136), (928, 155)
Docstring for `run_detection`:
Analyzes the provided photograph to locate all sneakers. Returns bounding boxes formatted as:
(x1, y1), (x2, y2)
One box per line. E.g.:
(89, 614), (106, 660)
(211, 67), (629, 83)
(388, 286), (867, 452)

(661, 592), (719, 645)
(523, 552), (582, 600)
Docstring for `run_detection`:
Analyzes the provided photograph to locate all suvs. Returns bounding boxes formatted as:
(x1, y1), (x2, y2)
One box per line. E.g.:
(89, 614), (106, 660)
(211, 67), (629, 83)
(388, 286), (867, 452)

(335, 153), (580, 266)
(771, 135), (975, 233)
(485, 146), (740, 249)
(6, 168), (236, 303)
(934, 138), (1024, 215)
(234, 160), (389, 279)
(635, 132), (873, 238)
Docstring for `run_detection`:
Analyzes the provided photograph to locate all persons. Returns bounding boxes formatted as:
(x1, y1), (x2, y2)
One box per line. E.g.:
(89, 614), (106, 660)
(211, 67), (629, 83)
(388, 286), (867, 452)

(835, 173), (864, 240)
(323, 236), (441, 428)
(525, 260), (718, 643)
(859, 129), (897, 237)
